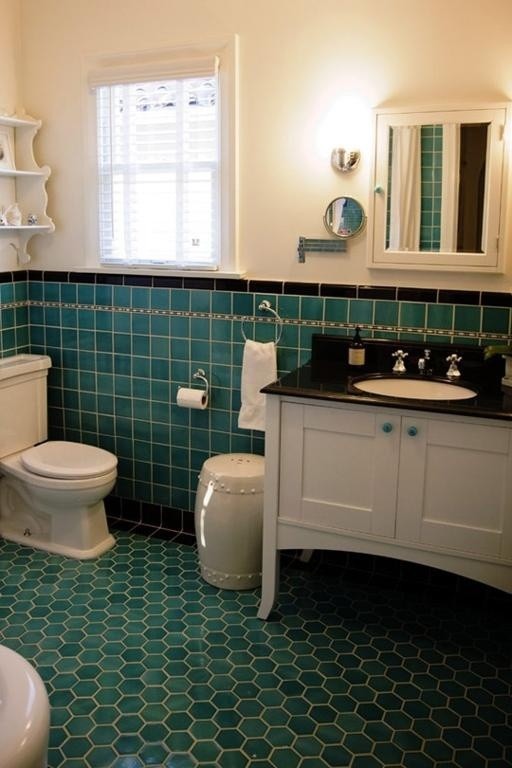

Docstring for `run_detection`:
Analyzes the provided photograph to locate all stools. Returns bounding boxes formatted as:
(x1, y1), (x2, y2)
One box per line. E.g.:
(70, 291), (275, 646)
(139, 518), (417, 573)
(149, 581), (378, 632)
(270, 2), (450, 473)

(193, 450), (267, 593)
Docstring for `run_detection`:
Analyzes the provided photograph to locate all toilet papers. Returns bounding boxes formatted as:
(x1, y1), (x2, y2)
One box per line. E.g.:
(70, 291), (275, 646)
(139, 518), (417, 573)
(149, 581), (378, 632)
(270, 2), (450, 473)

(176, 387), (208, 410)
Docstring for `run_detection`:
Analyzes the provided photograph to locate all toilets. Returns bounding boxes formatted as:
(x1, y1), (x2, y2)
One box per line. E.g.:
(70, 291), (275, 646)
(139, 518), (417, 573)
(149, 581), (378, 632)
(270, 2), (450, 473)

(0, 353), (118, 558)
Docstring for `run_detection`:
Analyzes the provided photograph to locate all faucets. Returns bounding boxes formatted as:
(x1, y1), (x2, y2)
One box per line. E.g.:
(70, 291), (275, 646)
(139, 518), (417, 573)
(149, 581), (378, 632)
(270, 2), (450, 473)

(418, 348), (433, 376)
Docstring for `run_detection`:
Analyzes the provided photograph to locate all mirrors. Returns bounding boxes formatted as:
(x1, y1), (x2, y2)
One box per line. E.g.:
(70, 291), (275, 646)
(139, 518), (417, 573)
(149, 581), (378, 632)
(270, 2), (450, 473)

(365, 99), (512, 276)
(322, 196), (367, 242)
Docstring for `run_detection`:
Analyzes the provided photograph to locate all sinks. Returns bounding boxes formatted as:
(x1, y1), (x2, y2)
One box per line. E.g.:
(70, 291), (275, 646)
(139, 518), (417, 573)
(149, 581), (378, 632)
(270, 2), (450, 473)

(351, 371), (479, 400)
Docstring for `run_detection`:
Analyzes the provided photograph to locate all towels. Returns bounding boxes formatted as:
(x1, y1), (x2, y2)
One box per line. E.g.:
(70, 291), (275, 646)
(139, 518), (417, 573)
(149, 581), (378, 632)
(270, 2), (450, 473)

(237, 338), (278, 435)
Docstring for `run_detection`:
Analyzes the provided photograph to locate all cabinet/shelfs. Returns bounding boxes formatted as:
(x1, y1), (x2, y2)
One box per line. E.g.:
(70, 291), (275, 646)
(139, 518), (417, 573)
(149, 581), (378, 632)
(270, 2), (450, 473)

(0, 105), (55, 265)
(257, 393), (512, 621)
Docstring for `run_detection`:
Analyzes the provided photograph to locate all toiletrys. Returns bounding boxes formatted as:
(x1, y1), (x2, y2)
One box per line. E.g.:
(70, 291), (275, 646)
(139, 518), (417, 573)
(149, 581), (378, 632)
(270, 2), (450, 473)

(347, 326), (366, 376)
